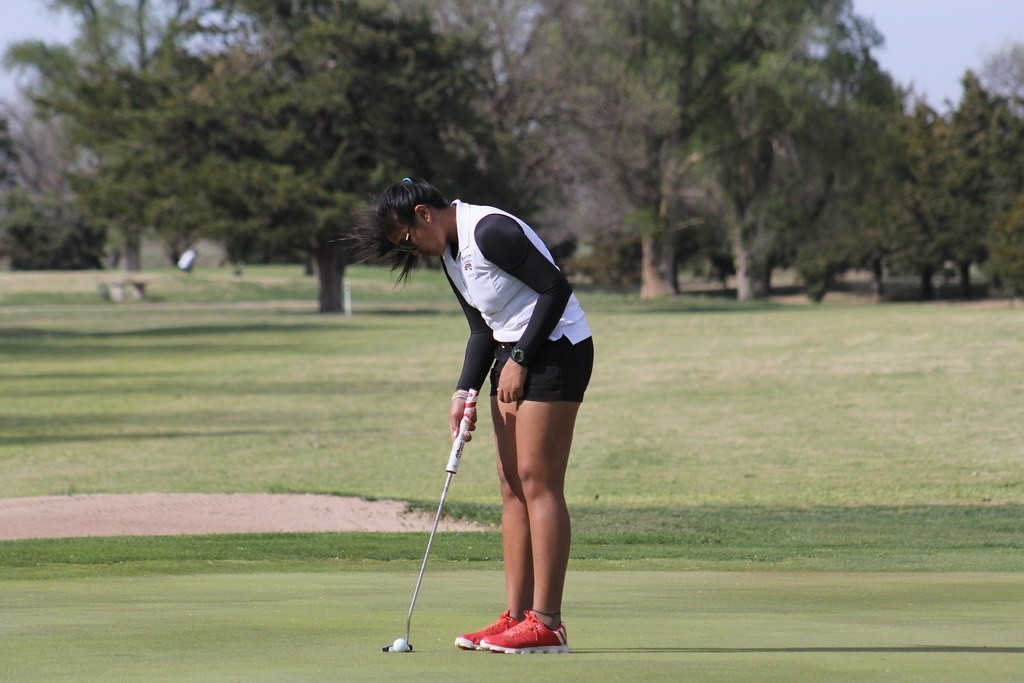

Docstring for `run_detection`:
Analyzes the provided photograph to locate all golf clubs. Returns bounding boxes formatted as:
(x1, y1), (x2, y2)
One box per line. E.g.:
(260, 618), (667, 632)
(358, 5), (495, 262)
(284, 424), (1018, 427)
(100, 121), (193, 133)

(380, 386), (481, 652)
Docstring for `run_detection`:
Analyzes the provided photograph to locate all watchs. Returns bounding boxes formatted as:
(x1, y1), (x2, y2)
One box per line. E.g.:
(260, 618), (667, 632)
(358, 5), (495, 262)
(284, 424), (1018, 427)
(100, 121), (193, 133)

(510, 349), (525, 362)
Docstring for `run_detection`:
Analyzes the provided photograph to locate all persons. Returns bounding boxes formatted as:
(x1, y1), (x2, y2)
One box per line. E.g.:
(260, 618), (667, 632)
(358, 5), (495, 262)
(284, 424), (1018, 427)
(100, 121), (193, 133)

(333, 178), (595, 655)
(177, 249), (197, 273)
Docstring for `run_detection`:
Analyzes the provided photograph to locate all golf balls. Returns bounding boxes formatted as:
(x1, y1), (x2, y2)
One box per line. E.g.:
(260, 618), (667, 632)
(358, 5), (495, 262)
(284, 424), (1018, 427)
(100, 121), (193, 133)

(393, 638), (407, 652)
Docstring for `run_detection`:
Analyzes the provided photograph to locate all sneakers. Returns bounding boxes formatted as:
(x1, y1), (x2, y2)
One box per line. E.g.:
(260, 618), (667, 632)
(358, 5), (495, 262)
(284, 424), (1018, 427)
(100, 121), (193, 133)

(454, 609), (522, 649)
(480, 610), (567, 654)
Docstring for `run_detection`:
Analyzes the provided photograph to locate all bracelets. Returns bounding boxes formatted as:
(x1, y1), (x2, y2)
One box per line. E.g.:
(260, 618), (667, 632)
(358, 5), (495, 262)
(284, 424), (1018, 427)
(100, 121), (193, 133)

(451, 391), (468, 402)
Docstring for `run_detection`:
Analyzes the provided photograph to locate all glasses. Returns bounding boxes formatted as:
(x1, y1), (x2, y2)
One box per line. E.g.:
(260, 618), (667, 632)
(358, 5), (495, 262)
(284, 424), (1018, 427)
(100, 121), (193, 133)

(396, 213), (419, 252)
